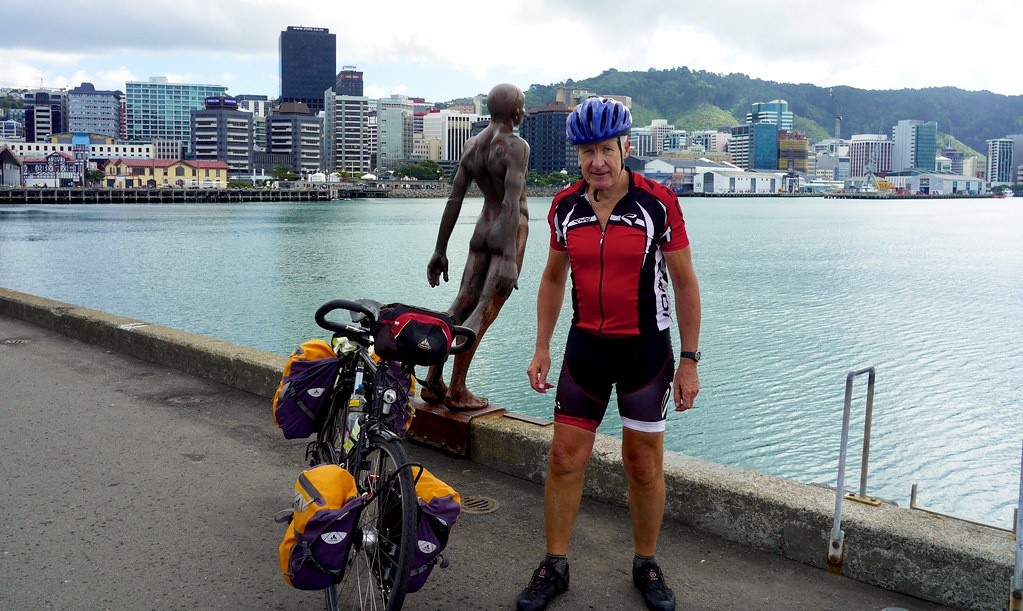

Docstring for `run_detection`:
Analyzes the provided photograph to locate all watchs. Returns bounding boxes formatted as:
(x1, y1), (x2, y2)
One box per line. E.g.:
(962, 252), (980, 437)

(681, 350), (702, 363)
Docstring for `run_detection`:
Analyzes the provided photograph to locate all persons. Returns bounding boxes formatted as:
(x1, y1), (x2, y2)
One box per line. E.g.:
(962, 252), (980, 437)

(419, 83), (529, 410)
(514, 96), (702, 611)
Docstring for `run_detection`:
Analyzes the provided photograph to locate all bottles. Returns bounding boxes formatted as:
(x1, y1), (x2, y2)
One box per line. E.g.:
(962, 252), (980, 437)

(345, 415), (369, 454)
(344, 384), (367, 443)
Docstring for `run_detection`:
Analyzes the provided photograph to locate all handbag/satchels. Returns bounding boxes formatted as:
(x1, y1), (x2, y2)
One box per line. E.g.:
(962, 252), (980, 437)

(374, 302), (454, 367)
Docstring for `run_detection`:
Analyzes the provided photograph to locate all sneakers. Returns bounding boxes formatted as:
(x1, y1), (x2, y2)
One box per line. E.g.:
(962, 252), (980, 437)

(517, 561), (570, 611)
(632, 560), (676, 611)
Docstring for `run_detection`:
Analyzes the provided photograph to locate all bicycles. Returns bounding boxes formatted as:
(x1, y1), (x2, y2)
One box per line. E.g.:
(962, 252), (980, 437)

(274, 298), (477, 611)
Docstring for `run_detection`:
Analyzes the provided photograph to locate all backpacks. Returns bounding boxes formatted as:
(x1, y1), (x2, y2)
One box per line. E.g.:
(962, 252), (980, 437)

(272, 338), (341, 440)
(279, 465), (366, 590)
(370, 353), (416, 438)
(378, 466), (462, 593)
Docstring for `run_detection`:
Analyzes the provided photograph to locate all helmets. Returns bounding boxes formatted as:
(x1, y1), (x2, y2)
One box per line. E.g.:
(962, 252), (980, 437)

(566, 96), (632, 145)
